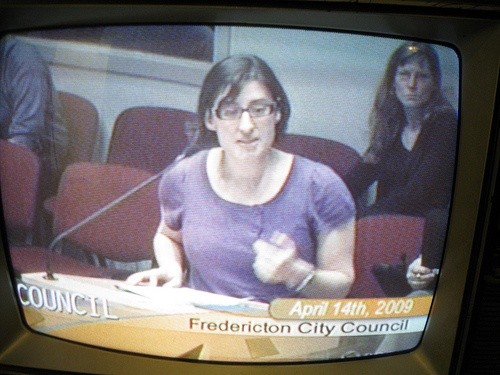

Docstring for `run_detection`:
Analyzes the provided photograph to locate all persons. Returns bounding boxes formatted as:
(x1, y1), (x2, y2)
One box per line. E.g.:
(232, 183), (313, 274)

(0, 30), (72, 247)
(120, 52), (355, 300)
(405, 249), (438, 293)
(340, 41), (462, 218)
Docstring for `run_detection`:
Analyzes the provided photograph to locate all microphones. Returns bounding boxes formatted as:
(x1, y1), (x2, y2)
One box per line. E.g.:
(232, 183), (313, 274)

(44, 143), (200, 281)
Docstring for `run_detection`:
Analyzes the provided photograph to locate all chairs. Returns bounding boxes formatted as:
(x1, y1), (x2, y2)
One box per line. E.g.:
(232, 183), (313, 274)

(0, 136), (38, 249)
(43, 93), (100, 197)
(341, 214), (428, 300)
(272, 136), (368, 208)
(52, 161), (168, 270)
(104, 108), (211, 179)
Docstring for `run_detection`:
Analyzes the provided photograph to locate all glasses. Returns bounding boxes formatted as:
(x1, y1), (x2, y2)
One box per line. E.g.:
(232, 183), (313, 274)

(210, 99), (279, 119)
(394, 68), (436, 83)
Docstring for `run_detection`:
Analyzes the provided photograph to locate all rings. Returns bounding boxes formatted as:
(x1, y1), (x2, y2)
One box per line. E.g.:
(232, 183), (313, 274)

(416, 272), (422, 282)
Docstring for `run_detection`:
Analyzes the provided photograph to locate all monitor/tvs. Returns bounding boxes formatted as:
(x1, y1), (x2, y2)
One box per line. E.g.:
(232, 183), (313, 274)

(2, 1), (499, 373)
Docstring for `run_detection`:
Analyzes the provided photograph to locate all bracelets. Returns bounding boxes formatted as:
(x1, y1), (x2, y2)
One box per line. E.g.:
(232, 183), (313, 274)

(293, 270), (315, 294)
(287, 261), (315, 290)
